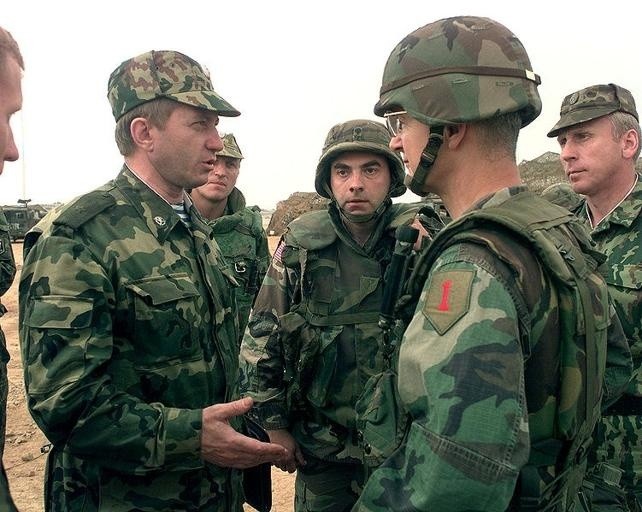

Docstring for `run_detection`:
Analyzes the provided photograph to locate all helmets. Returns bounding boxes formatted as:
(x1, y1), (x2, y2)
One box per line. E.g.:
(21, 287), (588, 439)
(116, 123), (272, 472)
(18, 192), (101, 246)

(374, 17), (542, 126)
(314, 119), (407, 197)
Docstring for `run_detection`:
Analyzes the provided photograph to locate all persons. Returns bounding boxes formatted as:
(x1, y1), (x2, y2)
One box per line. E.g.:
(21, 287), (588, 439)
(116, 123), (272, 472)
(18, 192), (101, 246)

(236, 118), (449, 512)
(17, 49), (293, 511)
(0, 26), (26, 512)
(183, 130), (271, 510)
(547, 82), (642, 512)
(352, 14), (636, 512)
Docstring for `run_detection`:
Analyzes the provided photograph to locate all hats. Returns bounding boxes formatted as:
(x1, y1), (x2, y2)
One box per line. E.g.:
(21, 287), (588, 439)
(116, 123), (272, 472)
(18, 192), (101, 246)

(547, 84), (638, 137)
(107, 50), (241, 122)
(216, 132), (244, 159)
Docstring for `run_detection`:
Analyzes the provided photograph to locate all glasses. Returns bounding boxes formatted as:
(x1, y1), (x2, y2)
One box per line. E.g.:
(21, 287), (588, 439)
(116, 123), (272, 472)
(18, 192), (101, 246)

(384, 110), (407, 137)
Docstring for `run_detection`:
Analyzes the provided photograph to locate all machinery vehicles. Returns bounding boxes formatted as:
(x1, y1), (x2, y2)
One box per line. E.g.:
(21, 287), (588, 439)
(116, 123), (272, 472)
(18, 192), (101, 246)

(0, 199), (50, 243)
